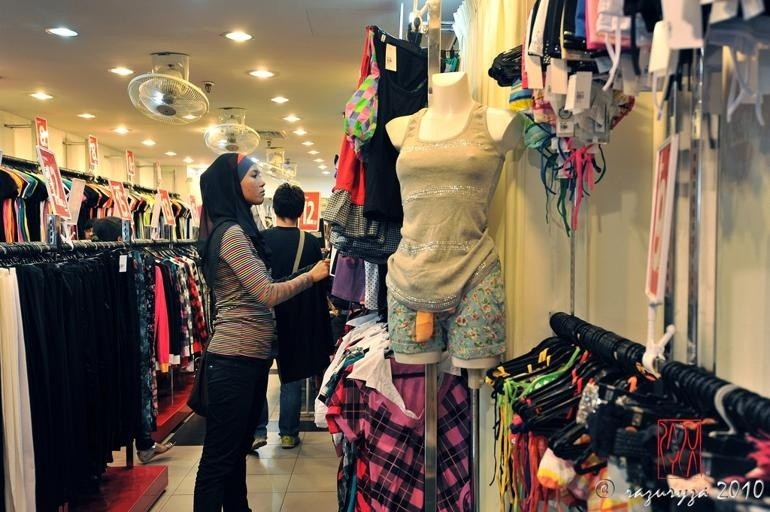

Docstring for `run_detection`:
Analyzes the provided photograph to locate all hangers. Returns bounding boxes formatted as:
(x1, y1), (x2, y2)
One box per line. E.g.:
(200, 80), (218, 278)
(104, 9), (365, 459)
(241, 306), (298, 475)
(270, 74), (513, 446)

(1, 238), (202, 271)
(0, 153), (181, 212)
(484, 311), (770, 475)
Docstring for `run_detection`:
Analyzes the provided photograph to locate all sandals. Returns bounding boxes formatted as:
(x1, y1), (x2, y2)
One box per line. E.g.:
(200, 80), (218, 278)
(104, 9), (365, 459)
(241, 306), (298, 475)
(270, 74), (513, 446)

(135, 440), (177, 463)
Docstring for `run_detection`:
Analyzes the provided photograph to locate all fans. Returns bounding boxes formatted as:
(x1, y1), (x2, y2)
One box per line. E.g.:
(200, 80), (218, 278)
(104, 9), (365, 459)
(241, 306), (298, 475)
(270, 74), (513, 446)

(250, 147), (294, 185)
(203, 107), (261, 156)
(269, 163), (304, 190)
(125, 53), (211, 127)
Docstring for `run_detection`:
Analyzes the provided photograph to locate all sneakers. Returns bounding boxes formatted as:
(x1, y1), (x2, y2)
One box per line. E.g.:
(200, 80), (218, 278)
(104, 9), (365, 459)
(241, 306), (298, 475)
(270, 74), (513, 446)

(279, 433), (301, 449)
(251, 436), (267, 449)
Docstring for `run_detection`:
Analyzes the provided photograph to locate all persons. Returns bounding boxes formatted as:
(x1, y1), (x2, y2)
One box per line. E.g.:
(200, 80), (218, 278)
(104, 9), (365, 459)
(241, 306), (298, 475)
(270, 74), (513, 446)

(93, 217), (176, 463)
(188, 153), (330, 512)
(251, 183), (323, 450)
(84, 220), (93, 239)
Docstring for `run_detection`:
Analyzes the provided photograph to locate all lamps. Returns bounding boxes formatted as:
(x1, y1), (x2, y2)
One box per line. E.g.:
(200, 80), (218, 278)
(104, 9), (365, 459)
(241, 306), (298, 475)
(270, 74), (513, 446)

(284, 115), (301, 121)
(109, 66), (132, 77)
(142, 139), (154, 146)
(303, 141), (313, 147)
(220, 28), (252, 43)
(296, 129), (306, 135)
(247, 69), (275, 78)
(165, 150), (176, 159)
(270, 95), (288, 105)
(112, 126), (131, 134)
